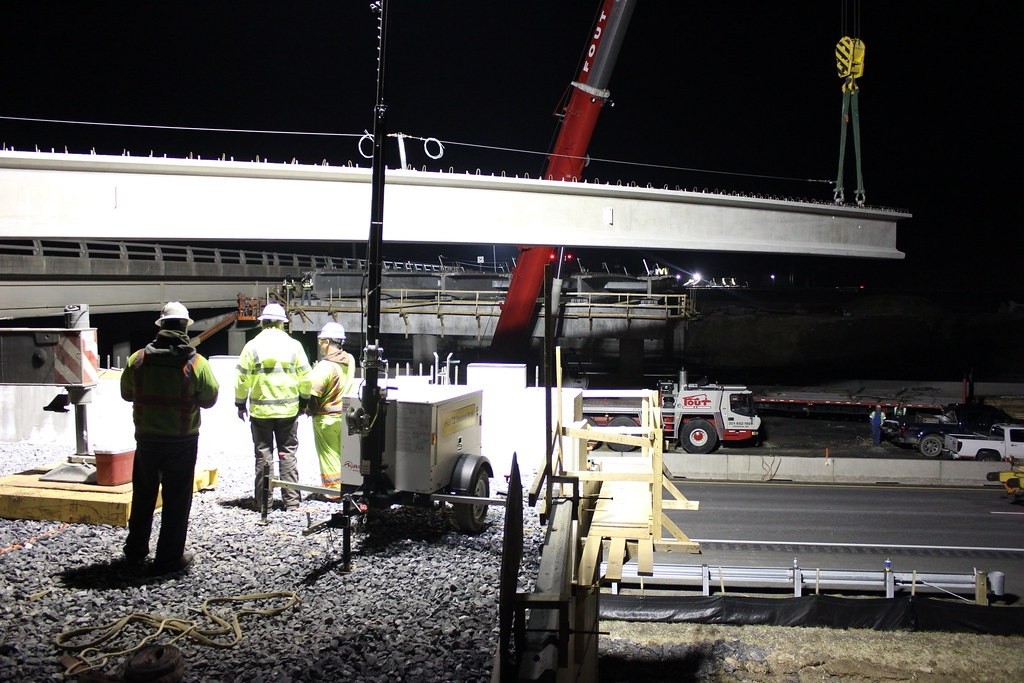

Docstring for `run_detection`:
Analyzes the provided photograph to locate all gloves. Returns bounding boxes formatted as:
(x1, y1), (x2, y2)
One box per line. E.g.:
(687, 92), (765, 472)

(238, 406), (248, 422)
(298, 407), (306, 417)
(306, 409), (313, 416)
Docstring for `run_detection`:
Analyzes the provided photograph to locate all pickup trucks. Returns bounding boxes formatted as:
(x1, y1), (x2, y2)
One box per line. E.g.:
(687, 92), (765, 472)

(940, 423), (1024, 463)
(879, 402), (1015, 458)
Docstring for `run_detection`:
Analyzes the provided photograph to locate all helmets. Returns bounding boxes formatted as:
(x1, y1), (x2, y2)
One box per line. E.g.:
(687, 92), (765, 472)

(317, 322), (346, 339)
(257, 303), (289, 322)
(155, 301), (194, 326)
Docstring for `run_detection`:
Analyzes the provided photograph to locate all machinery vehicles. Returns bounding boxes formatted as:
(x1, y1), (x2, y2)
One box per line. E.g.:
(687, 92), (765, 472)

(580, 365), (763, 453)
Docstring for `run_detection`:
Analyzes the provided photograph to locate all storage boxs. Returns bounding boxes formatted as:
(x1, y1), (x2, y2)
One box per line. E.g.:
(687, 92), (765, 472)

(93, 446), (137, 485)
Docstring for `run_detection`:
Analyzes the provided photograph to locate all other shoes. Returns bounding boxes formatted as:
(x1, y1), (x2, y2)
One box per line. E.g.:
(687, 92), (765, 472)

(127, 556), (154, 566)
(282, 506), (299, 510)
(307, 493), (341, 503)
(250, 501), (272, 513)
(154, 554), (194, 576)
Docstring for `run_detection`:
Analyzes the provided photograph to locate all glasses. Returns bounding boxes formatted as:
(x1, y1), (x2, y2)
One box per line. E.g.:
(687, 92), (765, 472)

(318, 339), (327, 345)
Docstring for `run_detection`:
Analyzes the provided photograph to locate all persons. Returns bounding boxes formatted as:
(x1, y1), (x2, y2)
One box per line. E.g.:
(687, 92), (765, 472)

(869, 405), (886, 444)
(894, 401), (907, 420)
(306, 323), (355, 499)
(731, 400), (740, 411)
(235, 303), (312, 513)
(120, 302), (219, 579)
